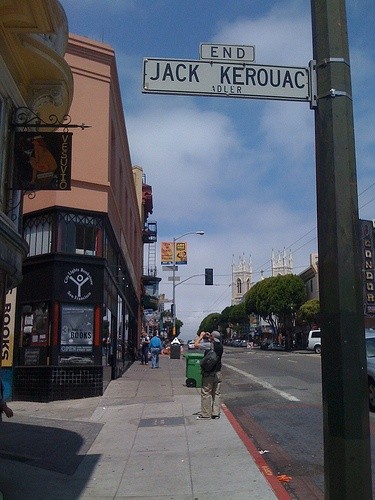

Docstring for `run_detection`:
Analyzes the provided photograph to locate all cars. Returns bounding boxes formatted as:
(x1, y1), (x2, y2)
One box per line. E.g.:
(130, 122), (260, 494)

(188, 341), (196, 350)
(365, 328), (375, 412)
(223, 338), (248, 347)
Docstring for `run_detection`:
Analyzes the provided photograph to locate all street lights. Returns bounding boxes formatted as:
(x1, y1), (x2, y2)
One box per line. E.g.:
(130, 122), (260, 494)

(173, 231), (205, 337)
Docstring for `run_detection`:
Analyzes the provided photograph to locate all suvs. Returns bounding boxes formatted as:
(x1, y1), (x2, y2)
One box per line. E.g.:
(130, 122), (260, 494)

(307, 329), (321, 354)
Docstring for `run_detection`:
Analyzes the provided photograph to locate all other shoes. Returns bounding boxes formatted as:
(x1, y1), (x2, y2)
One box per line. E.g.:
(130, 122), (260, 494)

(213, 416), (220, 419)
(196, 415), (211, 420)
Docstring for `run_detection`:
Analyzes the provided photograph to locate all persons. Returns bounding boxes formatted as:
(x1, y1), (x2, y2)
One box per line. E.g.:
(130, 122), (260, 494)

(0, 377), (14, 421)
(250, 337), (285, 351)
(195, 330), (224, 420)
(141, 332), (169, 370)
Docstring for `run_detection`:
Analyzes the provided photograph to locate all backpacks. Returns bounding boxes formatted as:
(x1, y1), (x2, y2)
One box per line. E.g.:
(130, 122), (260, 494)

(201, 349), (219, 372)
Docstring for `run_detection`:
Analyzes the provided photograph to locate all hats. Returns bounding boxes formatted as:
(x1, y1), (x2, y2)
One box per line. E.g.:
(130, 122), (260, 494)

(212, 331), (221, 342)
